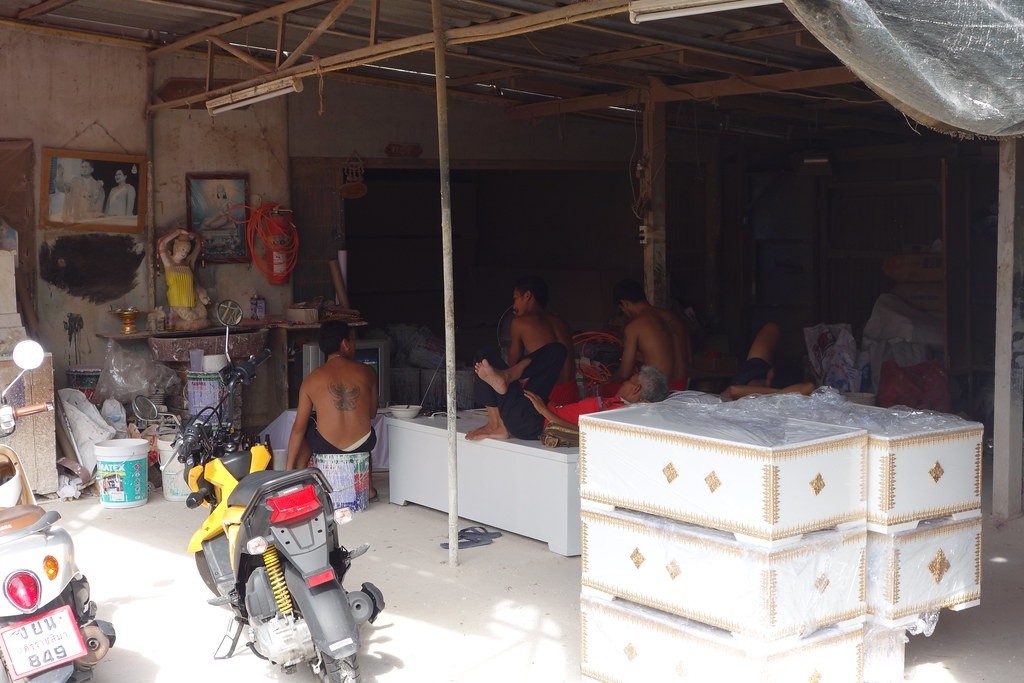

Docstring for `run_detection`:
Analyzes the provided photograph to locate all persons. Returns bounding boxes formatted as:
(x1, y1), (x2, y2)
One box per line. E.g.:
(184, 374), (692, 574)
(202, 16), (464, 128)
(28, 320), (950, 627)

(104, 167), (136, 217)
(463, 342), (672, 440)
(284, 320), (380, 503)
(158, 227), (208, 322)
(612, 279), (694, 393)
(200, 184), (233, 231)
(508, 275), (583, 408)
(54, 159), (105, 217)
(719, 321), (817, 400)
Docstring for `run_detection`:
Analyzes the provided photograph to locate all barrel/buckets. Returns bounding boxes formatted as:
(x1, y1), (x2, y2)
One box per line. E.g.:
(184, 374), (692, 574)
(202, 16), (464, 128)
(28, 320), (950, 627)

(186, 369), (222, 424)
(843, 392), (876, 406)
(369, 322), (475, 409)
(157, 434), (191, 501)
(317, 452), (369, 511)
(65, 367), (101, 406)
(94, 439), (150, 508)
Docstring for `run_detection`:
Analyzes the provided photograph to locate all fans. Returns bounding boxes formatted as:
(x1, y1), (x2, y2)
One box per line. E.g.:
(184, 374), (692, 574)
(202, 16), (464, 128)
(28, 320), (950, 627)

(496, 302), (564, 355)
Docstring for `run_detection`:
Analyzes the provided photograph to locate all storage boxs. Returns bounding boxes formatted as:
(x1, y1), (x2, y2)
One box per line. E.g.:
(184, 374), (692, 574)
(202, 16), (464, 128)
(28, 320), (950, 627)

(578, 406), (869, 549)
(856, 403), (984, 535)
(886, 254), (941, 283)
(693, 352), (739, 373)
(896, 285), (942, 311)
(579, 506), (867, 651)
(866, 517), (981, 629)
(580, 595), (866, 683)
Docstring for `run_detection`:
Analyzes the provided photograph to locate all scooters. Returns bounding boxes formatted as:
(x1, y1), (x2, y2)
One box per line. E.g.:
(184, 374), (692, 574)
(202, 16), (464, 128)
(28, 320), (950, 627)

(0, 340), (114, 683)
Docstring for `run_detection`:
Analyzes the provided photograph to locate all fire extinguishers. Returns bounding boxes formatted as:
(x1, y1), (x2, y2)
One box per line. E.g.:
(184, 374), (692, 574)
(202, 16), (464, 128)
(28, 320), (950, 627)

(266, 223), (289, 285)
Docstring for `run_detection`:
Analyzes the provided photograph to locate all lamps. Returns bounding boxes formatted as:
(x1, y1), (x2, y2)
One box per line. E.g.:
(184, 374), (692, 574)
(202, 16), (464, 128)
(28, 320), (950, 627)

(628, 0), (784, 24)
(206, 76), (304, 116)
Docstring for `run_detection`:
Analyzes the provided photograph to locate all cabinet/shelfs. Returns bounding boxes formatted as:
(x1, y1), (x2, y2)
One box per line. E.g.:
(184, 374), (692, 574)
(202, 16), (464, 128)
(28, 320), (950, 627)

(267, 320), (370, 415)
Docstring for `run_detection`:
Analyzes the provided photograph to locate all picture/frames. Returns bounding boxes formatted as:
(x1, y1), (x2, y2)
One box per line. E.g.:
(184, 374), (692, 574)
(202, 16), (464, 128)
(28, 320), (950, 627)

(38, 148), (147, 234)
(185, 172), (254, 264)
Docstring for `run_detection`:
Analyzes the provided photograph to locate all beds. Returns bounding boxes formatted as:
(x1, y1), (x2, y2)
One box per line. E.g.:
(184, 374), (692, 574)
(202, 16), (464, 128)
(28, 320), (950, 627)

(386, 409), (581, 557)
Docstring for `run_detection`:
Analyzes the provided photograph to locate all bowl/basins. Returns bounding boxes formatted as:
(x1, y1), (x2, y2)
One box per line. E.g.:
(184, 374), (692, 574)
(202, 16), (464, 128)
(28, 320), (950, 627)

(389, 405), (422, 419)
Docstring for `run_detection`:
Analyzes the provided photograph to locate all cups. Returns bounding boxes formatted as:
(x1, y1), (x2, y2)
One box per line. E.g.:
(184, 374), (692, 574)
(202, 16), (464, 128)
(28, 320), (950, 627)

(190, 349), (203, 371)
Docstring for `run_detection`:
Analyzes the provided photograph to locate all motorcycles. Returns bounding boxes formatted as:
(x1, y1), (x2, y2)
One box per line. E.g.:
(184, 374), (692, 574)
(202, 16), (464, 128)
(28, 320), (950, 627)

(129, 297), (385, 683)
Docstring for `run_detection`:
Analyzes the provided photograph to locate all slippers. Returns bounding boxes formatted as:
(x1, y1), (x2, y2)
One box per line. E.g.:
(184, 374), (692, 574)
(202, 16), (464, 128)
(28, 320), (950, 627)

(440, 536), (491, 549)
(369, 490), (378, 502)
(458, 526), (502, 539)
